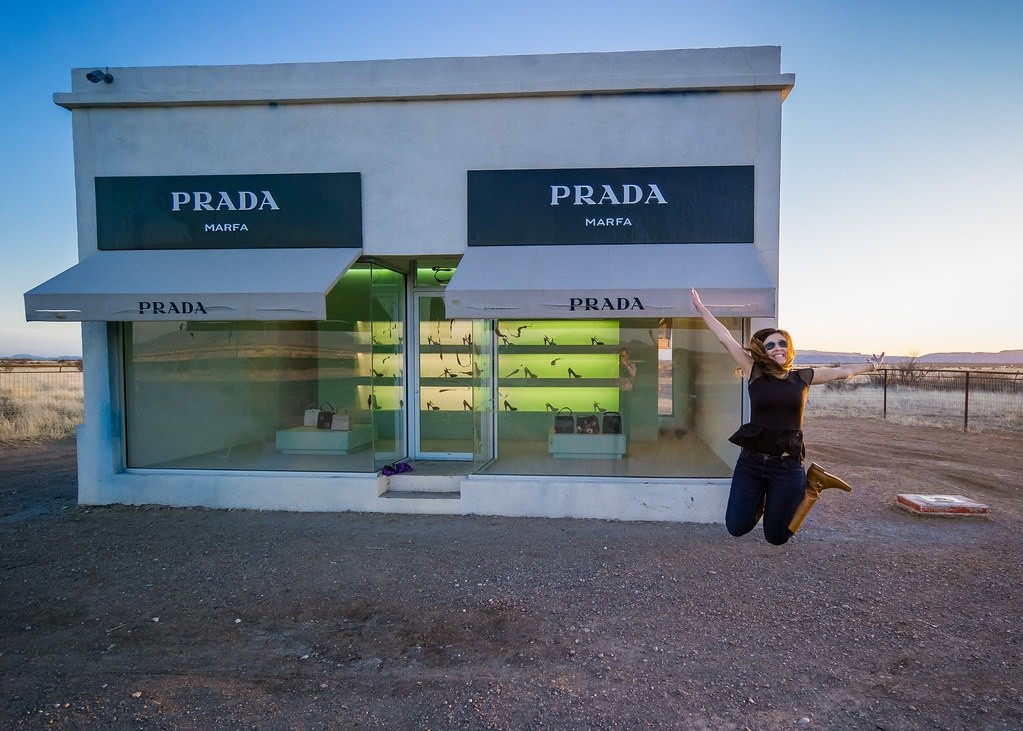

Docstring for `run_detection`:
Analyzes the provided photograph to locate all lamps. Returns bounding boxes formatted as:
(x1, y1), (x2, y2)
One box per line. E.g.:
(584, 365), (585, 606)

(86, 70), (113, 84)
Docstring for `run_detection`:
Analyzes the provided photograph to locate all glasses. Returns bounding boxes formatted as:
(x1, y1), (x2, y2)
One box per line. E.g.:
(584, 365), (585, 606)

(764, 340), (787, 350)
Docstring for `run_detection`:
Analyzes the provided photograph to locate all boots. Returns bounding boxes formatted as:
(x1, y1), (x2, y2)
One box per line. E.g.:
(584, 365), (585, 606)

(756, 462), (852, 535)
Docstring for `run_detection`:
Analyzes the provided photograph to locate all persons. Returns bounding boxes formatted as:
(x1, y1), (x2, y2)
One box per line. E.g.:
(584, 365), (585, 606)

(618, 347), (637, 457)
(690, 288), (886, 545)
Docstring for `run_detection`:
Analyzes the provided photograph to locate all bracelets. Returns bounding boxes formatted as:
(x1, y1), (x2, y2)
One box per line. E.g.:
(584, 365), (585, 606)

(867, 360), (878, 372)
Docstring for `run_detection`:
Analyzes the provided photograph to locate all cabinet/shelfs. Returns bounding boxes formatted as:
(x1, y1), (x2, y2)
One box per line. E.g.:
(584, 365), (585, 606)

(355, 340), (619, 441)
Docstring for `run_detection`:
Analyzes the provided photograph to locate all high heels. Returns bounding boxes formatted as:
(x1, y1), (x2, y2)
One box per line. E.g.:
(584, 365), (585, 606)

(399, 337), (403, 344)
(463, 400), (472, 410)
(463, 335), (472, 345)
(400, 400), (403, 410)
(503, 337), (513, 345)
(399, 370), (403, 377)
(568, 367), (582, 378)
(544, 334), (557, 345)
(524, 367), (538, 378)
(427, 400), (439, 410)
(593, 401), (608, 413)
(545, 401), (559, 412)
(368, 395), (382, 409)
(444, 366), (457, 377)
(428, 334), (440, 345)
(370, 369), (383, 377)
(591, 335), (604, 345)
(373, 335), (383, 344)
(504, 400), (517, 411)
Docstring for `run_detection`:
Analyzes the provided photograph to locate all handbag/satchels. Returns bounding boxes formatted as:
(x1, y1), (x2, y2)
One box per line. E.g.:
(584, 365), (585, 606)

(303, 402), (351, 431)
(554, 407), (622, 434)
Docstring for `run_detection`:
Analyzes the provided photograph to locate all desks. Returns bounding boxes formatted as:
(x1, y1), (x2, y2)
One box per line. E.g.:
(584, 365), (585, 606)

(547, 424), (628, 460)
(275, 422), (377, 455)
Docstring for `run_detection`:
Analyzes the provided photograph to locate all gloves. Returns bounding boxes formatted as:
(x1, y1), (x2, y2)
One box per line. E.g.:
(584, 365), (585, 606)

(865, 352), (885, 374)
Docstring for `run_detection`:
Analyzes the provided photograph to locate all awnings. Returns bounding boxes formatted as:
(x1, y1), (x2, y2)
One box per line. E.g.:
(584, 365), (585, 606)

(444, 245), (777, 318)
(23, 248), (362, 322)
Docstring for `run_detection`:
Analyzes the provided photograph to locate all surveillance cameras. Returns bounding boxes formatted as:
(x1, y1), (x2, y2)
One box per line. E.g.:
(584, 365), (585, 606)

(86, 70), (106, 83)
